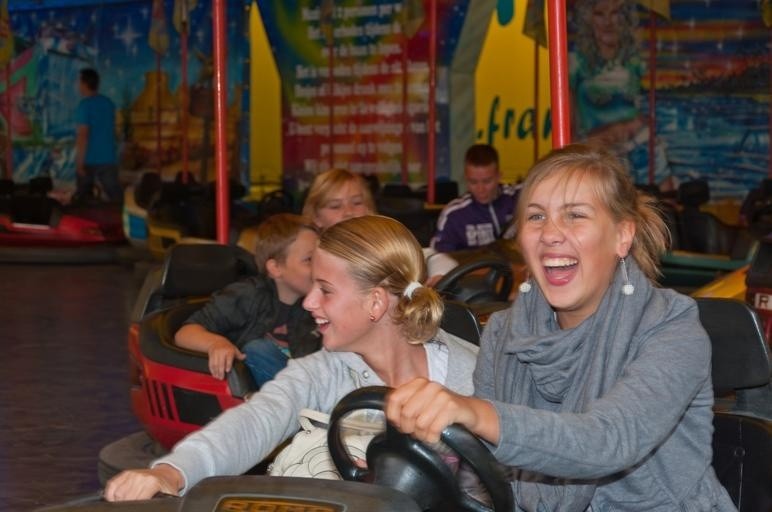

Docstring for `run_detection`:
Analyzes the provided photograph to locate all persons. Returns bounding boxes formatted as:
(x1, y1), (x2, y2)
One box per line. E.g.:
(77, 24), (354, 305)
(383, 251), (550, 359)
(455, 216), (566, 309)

(384, 143), (739, 512)
(566, 1), (675, 192)
(102, 214), (493, 510)
(73, 68), (124, 206)
(172, 212), (322, 389)
(431, 144), (527, 255)
(301, 168), (458, 287)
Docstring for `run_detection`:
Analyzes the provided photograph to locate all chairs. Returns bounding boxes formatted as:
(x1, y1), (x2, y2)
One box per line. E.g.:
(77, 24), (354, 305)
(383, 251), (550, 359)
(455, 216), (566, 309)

(675, 181), (730, 253)
(163, 243), (261, 329)
(693, 297), (772, 512)
